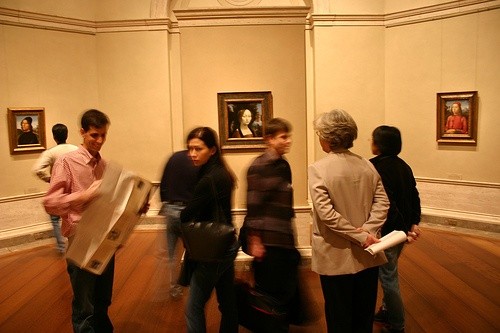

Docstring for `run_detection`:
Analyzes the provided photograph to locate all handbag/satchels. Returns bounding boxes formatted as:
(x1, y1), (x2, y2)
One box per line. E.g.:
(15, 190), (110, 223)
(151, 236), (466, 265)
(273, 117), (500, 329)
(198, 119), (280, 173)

(237, 216), (249, 252)
(233, 256), (290, 333)
(180, 176), (235, 263)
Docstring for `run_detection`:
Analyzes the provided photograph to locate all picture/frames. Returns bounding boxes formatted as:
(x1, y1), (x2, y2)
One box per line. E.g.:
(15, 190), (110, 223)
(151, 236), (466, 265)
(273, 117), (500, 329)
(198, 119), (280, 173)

(435, 90), (478, 146)
(217, 90), (273, 154)
(6, 106), (47, 155)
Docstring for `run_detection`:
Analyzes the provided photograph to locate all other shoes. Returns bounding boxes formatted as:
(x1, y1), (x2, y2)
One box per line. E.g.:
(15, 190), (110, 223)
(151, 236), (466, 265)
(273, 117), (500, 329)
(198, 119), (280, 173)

(374, 305), (386, 321)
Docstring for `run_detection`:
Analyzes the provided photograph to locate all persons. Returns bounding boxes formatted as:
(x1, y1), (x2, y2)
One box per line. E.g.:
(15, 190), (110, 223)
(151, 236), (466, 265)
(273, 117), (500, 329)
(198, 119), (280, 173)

(308, 108), (390, 333)
(369, 125), (421, 332)
(32, 109), (150, 333)
(160, 127), (240, 331)
(239, 118), (300, 332)
